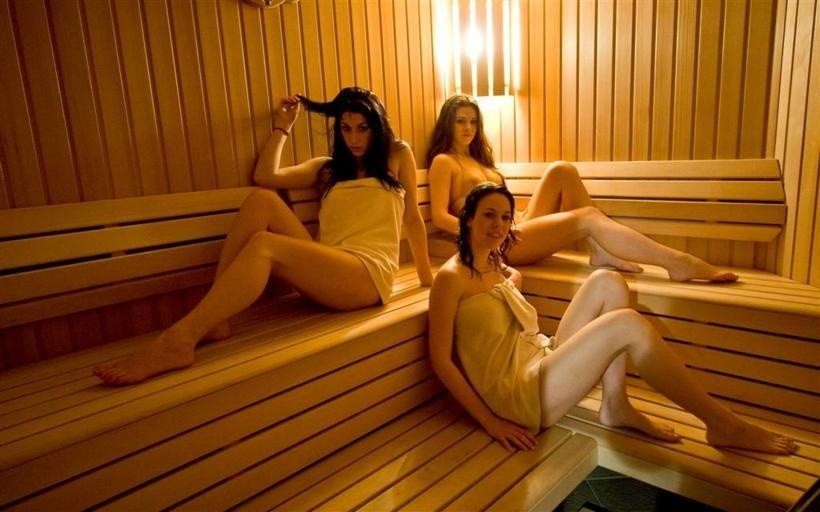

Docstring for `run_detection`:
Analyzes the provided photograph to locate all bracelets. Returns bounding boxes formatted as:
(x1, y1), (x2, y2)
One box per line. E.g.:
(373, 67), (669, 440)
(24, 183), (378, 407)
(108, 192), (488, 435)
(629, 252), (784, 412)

(272, 124), (293, 137)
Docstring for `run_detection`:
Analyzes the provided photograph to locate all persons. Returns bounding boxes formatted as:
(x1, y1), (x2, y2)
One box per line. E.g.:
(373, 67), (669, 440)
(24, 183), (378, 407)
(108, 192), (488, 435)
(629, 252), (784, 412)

(89, 85), (437, 385)
(424, 96), (740, 286)
(427, 179), (803, 459)
